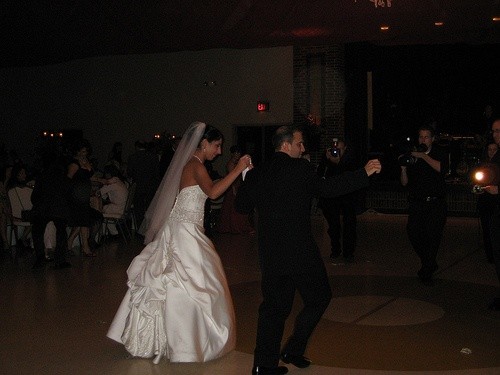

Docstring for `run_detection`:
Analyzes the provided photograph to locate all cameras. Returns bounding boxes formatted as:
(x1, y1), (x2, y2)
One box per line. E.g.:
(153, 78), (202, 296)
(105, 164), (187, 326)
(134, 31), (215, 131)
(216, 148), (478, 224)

(328, 137), (340, 157)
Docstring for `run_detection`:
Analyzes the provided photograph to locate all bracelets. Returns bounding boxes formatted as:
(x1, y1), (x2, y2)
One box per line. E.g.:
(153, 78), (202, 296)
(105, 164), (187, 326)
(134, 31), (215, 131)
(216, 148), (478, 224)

(96, 177), (101, 183)
(494, 186), (498, 194)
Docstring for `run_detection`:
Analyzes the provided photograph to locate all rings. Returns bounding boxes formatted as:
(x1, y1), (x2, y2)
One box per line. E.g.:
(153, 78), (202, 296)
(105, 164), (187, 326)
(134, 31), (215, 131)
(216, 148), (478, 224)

(246, 156), (249, 159)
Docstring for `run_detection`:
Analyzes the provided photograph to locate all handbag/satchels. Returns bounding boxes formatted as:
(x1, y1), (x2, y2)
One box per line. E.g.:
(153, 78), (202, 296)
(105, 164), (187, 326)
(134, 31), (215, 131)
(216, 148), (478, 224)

(21, 210), (32, 221)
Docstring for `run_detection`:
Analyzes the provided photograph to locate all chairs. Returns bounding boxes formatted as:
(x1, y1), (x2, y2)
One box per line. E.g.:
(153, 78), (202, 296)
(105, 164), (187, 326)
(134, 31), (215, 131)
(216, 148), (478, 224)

(0, 166), (225, 257)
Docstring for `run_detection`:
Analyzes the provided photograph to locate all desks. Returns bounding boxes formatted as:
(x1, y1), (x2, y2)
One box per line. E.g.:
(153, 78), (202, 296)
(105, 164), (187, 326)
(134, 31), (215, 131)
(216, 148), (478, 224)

(68, 191), (103, 247)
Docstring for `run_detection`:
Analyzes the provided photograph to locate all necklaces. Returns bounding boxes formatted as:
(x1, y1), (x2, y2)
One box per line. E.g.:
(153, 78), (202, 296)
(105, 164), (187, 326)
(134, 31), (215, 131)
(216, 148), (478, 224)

(193, 155), (204, 166)
(80, 158), (89, 167)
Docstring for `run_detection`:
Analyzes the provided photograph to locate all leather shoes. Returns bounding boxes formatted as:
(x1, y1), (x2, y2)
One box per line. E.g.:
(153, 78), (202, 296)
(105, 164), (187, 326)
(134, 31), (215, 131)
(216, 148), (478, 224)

(252, 364), (290, 375)
(281, 350), (310, 369)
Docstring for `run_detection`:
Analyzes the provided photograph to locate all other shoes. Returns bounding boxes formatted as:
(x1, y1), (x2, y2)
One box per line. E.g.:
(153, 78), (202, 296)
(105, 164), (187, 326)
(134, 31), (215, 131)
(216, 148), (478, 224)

(330, 245), (354, 263)
(418, 263), (439, 275)
(80, 249), (95, 256)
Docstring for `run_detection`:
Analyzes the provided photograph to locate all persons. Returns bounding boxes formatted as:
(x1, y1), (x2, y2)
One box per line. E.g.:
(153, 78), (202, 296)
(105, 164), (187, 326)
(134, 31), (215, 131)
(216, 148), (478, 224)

(242, 125), (381, 375)
(398, 126), (449, 278)
(478, 120), (500, 269)
(217, 145), (256, 233)
(314, 140), (357, 263)
(0, 135), (224, 270)
(107, 122), (252, 365)
